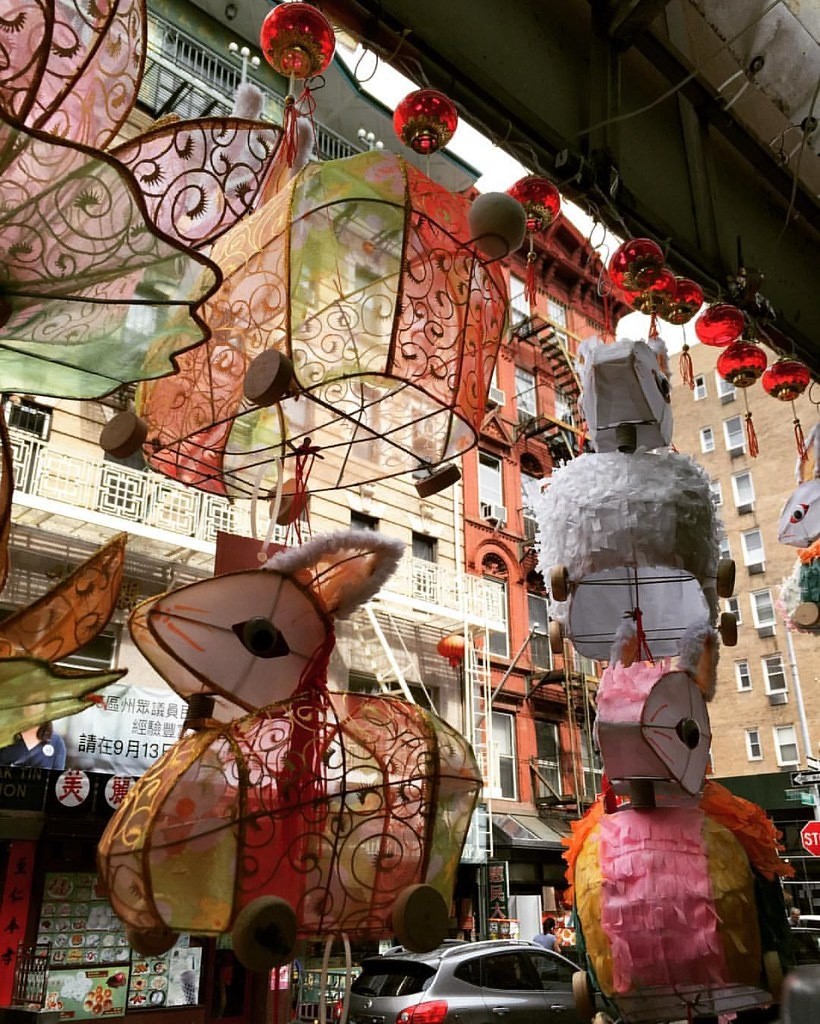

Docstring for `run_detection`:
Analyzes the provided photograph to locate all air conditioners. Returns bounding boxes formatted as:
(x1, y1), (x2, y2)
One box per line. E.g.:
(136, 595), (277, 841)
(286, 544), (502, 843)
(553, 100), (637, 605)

(486, 386), (506, 408)
(482, 504), (506, 523)
(3, 400), (50, 440)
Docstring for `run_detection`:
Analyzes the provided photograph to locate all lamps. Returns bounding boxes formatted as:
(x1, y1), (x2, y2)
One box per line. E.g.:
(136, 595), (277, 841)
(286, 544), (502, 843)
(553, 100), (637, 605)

(225, 4), (237, 20)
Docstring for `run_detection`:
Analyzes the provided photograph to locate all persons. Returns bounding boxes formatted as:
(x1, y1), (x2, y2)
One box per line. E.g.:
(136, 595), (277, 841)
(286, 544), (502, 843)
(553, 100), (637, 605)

(533, 916), (561, 979)
(787, 907), (801, 927)
(0, 677), (66, 770)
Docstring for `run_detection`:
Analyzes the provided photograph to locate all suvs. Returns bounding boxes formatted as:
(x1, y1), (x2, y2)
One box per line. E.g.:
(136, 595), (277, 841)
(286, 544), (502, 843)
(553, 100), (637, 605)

(334, 939), (618, 1024)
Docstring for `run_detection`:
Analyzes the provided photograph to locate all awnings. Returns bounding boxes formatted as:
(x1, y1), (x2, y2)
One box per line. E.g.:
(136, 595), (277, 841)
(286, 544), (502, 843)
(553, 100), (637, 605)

(485, 813), (582, 851)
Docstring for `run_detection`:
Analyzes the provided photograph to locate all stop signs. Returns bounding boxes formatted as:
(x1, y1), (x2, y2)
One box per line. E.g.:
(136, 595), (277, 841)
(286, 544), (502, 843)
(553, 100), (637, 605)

(801, 821), (820, 856)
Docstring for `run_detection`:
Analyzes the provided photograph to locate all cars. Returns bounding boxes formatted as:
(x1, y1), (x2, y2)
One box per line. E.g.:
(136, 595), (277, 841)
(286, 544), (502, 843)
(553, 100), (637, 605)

(788, 915), (820, 966)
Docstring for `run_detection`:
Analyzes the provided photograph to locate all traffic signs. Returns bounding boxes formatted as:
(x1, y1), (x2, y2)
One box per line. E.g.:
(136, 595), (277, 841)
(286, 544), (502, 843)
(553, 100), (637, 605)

(789, 756), (820, 788)
